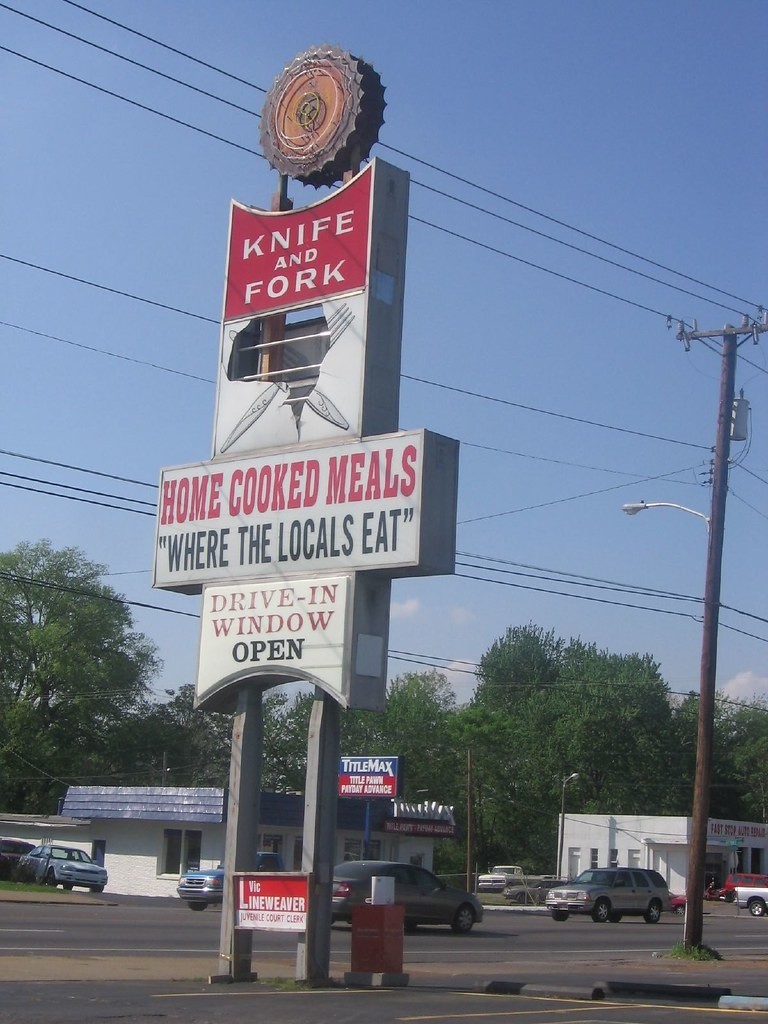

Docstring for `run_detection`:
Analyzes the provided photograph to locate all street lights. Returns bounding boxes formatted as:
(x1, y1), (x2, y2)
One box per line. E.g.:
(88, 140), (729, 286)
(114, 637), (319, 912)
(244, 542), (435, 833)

(558, 772), (579, 881)
(622, 499), (724, 944)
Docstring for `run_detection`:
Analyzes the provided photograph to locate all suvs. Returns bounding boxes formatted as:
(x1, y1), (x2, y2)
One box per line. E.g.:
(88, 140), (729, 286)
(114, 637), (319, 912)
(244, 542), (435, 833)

(178, 852), (285, 911)
(545, 866), (669, 924)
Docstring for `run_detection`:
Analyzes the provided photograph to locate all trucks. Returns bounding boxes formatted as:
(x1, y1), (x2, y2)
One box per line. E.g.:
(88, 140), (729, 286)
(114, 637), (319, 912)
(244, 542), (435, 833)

(477, 865), (558, 893)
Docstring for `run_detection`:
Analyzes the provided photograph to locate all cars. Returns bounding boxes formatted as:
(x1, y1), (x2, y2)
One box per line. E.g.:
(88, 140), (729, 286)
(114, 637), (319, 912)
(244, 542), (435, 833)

(17, 844), (108, 893)
(330, 860), (484, 936)
(667, 890), (688, 917)
(0, 838), (36, 880)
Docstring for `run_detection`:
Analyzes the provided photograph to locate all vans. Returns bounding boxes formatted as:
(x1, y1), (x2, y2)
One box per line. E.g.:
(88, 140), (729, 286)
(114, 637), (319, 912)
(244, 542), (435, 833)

(723, 873), (768, 903)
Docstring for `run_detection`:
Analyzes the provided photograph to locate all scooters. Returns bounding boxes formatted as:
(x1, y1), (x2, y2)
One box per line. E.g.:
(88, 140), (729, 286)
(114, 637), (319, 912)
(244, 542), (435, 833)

(703, 875), (734, 903)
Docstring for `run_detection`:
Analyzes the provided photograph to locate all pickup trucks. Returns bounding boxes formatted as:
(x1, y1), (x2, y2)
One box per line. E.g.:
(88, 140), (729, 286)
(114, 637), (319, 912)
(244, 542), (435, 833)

(733, 886), (768, 917)
